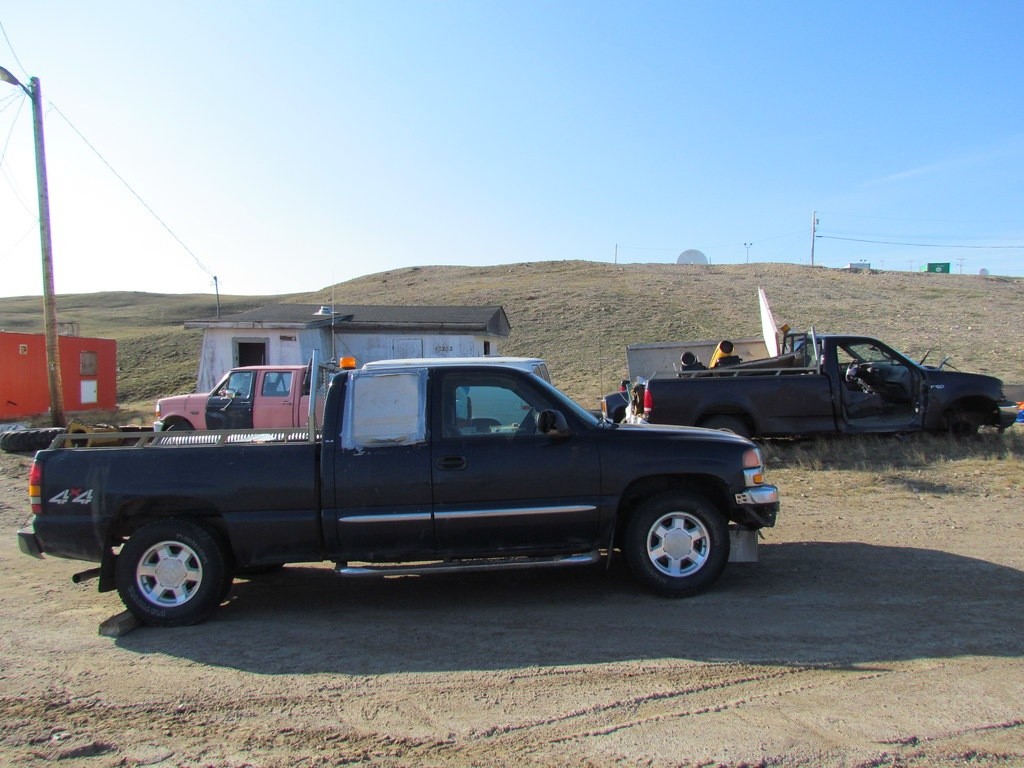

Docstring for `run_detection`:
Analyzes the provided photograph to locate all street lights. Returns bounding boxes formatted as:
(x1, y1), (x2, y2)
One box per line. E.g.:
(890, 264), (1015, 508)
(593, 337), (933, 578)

(0, 65), (67, 427)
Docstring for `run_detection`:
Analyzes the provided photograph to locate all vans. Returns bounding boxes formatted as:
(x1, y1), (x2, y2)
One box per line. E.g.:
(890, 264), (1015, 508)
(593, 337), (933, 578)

(351, 357), (553, 446)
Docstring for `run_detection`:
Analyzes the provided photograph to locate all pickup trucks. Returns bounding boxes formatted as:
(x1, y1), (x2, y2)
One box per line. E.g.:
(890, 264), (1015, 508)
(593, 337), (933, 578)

(16, 365), (781, 627)
(150, 364), (324, 432)
(598, 330), (1019, 441)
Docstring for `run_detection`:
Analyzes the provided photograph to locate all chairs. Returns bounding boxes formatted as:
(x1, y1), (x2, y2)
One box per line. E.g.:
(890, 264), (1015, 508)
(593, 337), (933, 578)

(839, 373), (881, 415)
(265, 383), (277, 395)
